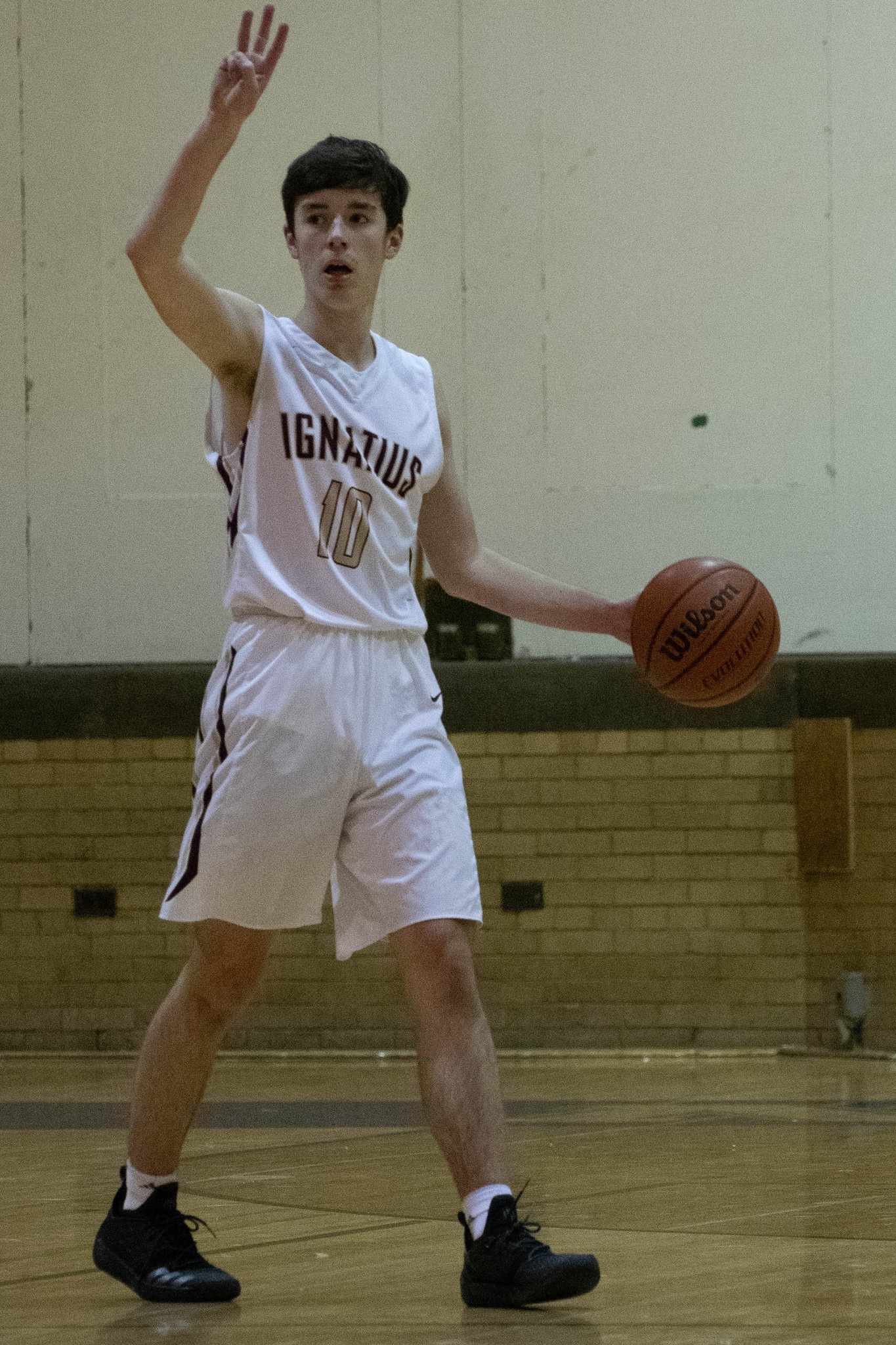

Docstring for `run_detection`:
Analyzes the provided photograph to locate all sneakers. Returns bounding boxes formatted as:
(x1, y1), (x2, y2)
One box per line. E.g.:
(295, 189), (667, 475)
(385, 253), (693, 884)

(457, 1179), (601, 1308)
(92, 1165), (241, 1303)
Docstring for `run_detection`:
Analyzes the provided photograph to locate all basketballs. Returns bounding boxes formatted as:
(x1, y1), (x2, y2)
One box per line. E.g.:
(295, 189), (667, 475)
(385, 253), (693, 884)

(630, 554), (785, 712)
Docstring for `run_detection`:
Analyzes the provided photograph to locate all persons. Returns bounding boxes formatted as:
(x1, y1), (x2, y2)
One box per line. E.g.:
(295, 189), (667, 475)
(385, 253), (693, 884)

(91, 3), (639, 1306)
